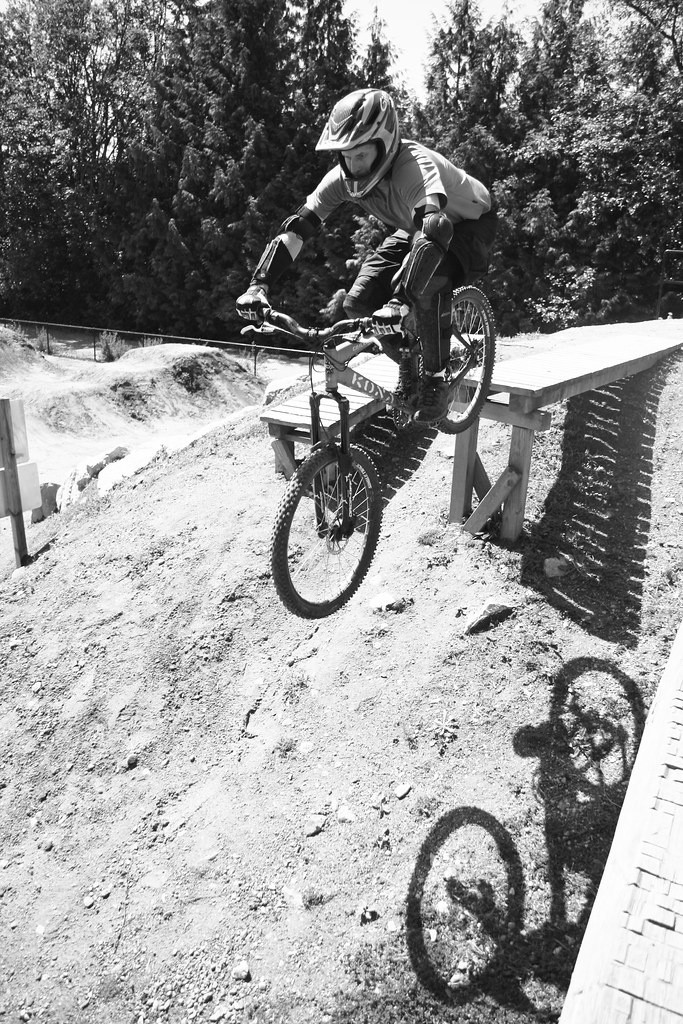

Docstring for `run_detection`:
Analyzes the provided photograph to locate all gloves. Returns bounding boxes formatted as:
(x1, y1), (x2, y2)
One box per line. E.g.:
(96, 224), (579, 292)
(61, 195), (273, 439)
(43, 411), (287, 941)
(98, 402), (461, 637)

(234, 280), (272, 321)
(372, 297), (413, 344)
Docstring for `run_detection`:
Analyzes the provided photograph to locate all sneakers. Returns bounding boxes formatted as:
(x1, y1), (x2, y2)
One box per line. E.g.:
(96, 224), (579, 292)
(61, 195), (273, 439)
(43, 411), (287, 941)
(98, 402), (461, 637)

(413, 367), (454, 423)
(395, 340), (424, 399)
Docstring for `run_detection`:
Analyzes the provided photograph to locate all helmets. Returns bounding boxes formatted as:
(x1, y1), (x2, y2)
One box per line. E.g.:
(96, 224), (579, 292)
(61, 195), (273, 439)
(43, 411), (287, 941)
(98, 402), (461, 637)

(314, 88), (401, 199)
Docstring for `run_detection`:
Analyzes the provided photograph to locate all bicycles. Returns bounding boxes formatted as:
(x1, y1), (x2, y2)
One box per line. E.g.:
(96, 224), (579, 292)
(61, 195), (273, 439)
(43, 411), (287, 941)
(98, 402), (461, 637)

(238, 252), (498, 620)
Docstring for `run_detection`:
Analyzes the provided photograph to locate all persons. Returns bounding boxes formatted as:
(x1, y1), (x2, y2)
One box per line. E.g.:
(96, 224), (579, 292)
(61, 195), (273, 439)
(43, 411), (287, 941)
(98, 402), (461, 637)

(235, 89), (500, 423)
